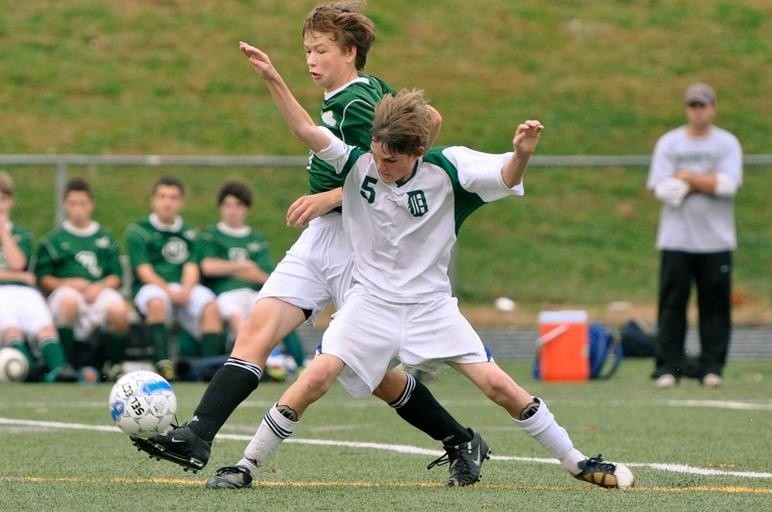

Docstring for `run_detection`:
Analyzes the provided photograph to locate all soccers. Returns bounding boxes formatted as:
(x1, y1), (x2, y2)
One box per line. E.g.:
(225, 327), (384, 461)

(0, 348), (31, 383)
(266, 348), (299, 380)
(109, 371), (177, 439)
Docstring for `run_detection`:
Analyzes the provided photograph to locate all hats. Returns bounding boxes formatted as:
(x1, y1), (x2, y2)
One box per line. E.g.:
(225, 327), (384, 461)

(684, 81), (716, 107)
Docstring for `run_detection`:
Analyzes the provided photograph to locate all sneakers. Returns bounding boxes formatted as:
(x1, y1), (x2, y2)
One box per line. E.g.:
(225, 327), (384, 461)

(53, 368), (76, 382)
(443, 425), (492, 488)
(159, 365), (178, 384)
(568, 456), (635, 490)
(127, 420), (212, 474)
(703, 373), (723, 389)
(655, 374), (678, 389)
(204, 465), (252, 490)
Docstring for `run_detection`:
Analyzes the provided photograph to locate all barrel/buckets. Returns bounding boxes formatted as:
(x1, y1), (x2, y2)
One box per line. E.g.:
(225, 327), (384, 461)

(535, 309), (590, 382)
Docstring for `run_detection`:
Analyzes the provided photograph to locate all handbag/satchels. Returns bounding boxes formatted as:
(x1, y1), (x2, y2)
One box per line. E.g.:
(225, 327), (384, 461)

(533, 323), (623, 381)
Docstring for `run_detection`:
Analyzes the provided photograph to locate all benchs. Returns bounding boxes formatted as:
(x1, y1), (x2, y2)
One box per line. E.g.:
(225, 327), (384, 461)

(25, 311), (159, 381)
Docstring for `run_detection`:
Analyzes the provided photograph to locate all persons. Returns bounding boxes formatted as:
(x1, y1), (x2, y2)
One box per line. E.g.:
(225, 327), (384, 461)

(127, 1), (492, 490)
(647, 83), (744, 388)
(206, 40), (634, 490)
